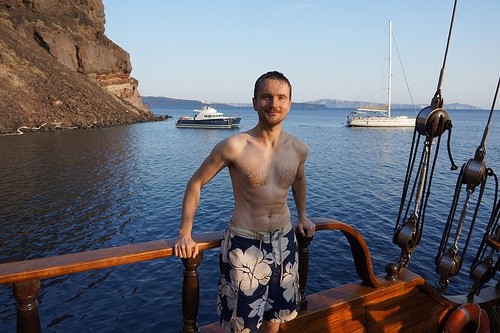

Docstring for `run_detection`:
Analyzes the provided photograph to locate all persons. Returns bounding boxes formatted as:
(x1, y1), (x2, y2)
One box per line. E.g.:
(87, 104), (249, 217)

(175, 70), (316, 333)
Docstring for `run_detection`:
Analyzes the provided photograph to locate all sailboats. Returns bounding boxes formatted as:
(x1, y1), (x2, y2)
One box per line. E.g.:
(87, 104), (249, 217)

(343, 18), (417, 127)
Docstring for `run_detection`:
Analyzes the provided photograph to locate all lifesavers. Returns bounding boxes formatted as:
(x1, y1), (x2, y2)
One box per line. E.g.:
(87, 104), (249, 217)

(443, 302), (494, 333)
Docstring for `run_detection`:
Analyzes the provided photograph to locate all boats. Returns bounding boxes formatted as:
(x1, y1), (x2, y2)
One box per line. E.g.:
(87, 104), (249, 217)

(175, 101), (240, 129)
